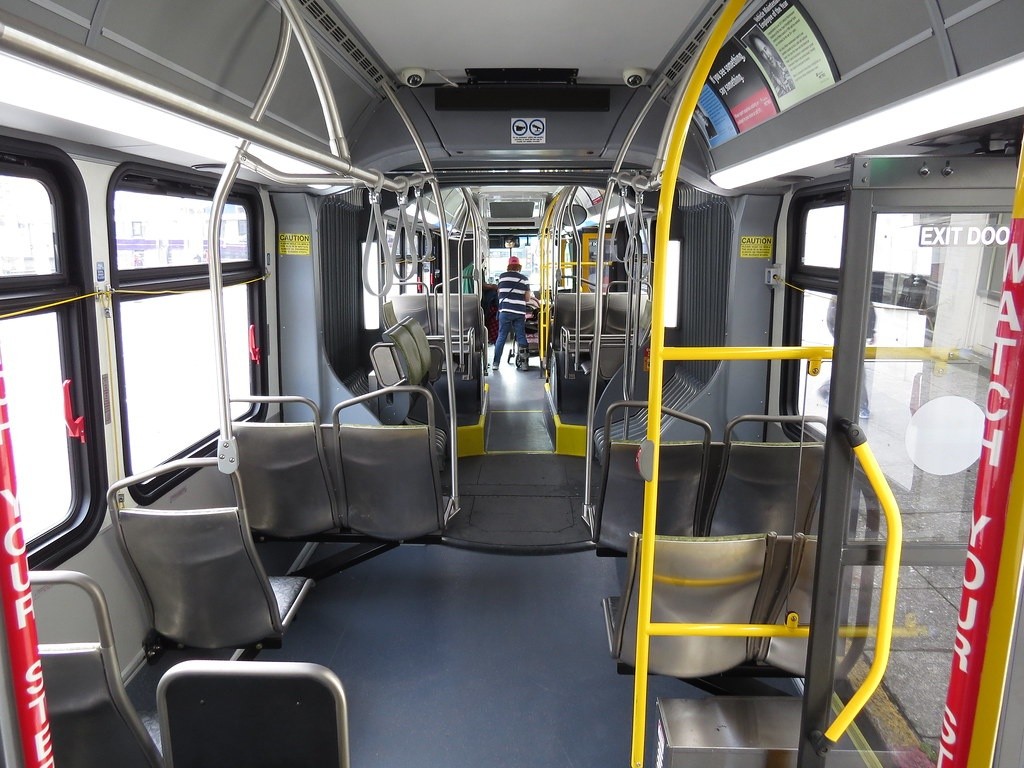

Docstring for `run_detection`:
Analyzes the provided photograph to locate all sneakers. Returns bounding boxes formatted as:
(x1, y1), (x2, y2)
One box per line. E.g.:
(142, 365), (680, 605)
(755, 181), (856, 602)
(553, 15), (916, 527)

(859, 406), (871, 419)
(818, 390), (829, 404)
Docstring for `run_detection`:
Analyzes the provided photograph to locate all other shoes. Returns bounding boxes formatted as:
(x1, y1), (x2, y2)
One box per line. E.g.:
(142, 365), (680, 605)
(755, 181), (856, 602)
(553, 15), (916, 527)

(492, 365), (499, 369)
(521, 365), (528, 370)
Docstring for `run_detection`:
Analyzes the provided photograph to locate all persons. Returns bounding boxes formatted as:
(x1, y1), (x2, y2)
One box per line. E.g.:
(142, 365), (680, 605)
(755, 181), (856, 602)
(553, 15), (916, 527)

(491, 256), (531, 371)
(813, 294), (877, 420)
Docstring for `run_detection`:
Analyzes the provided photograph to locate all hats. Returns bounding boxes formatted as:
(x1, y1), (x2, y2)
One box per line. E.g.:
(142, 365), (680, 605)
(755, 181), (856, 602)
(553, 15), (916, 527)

(509, 256), (519, 264)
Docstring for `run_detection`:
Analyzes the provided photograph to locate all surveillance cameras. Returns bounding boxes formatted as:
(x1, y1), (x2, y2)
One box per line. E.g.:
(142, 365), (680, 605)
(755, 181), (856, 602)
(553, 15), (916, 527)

(401, 68), (425, 87)
(623, 68), (646, 88)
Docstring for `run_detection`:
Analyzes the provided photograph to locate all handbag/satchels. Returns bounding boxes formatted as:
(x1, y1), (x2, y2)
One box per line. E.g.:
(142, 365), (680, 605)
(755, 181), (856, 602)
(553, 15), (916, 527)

(496, 311), (499, 320)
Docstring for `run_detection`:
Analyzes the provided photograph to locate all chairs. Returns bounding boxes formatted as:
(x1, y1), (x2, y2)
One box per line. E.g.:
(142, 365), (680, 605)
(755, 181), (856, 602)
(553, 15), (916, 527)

(22, 274), (826, 768)
